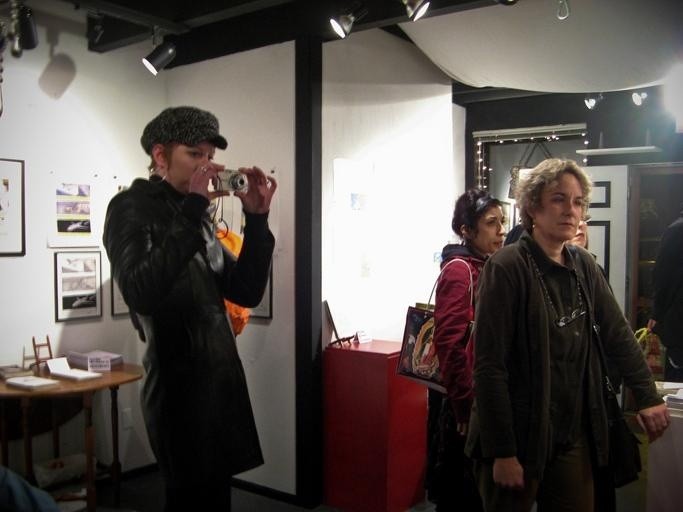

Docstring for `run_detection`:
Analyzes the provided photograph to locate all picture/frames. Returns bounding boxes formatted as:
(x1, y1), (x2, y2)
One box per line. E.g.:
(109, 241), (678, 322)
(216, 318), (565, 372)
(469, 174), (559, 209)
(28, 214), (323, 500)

(110, 279), (128, 318)
(52, 249), (103, 326)
(0, 160), (24, 257)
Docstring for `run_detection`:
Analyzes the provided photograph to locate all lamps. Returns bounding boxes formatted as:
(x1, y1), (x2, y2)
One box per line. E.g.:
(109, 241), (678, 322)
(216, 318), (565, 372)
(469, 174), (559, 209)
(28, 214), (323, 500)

(328, 13), (358, 40)
(399, 2), (433, 23)
(141, 23), (177, 79)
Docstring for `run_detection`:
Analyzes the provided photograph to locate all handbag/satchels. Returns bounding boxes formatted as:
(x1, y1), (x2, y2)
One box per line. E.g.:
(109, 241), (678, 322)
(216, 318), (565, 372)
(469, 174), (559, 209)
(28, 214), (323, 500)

(395, 302), (477, 397)
(600, 384), (645, 490)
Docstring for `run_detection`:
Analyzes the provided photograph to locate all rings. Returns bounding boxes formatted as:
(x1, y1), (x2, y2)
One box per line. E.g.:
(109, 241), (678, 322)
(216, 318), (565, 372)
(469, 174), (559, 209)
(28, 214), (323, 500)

(200, 166), (204, 170)
(202, 168), (208, 173)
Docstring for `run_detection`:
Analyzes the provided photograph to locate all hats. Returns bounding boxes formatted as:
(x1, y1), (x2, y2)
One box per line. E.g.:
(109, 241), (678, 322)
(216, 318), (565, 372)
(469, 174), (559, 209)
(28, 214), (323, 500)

(138, 105), (229, 153)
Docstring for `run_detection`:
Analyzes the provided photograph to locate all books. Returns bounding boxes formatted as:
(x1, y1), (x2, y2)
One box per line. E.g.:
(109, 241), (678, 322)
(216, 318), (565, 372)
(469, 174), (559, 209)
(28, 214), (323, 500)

(653, 380), (682, 415)
(1, 348), (123, 392)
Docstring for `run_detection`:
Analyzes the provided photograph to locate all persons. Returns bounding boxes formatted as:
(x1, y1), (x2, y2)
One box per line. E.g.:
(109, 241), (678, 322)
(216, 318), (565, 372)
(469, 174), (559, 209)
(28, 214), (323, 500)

(564, 216), (625, 398)
(645, 215), (683, 381)
(461, 155), (671, 510)
(425, 184), (507, 512)
(100, 104), (278, 512)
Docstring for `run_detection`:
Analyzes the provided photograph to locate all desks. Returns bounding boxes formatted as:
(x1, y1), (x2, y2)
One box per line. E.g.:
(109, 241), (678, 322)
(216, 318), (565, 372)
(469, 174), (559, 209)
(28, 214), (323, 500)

(324, 331), (428, 509)
(0, 362), (145, 504)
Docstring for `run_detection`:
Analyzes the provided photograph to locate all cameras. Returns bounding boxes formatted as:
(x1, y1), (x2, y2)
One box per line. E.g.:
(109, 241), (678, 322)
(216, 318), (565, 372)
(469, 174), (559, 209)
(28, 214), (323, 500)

(214, 167), (251, 193)
